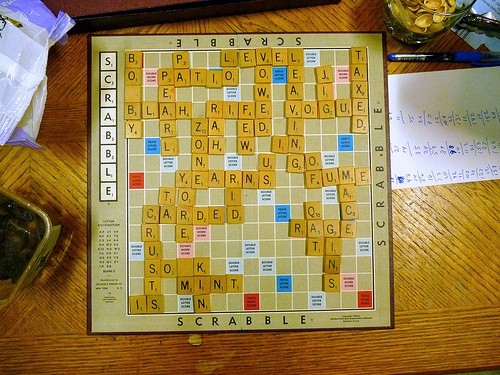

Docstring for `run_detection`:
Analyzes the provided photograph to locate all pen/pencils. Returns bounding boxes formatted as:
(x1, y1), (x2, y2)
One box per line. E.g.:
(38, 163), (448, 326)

(388, 51), (500, 67)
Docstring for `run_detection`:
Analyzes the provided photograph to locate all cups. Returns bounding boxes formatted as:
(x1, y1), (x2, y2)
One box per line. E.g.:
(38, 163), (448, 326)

(2, 185), (62, 306)
(382, 0), (476, 49)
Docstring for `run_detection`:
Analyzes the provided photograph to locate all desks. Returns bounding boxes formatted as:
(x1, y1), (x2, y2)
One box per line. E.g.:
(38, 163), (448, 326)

(0, 1), (500, 375)
